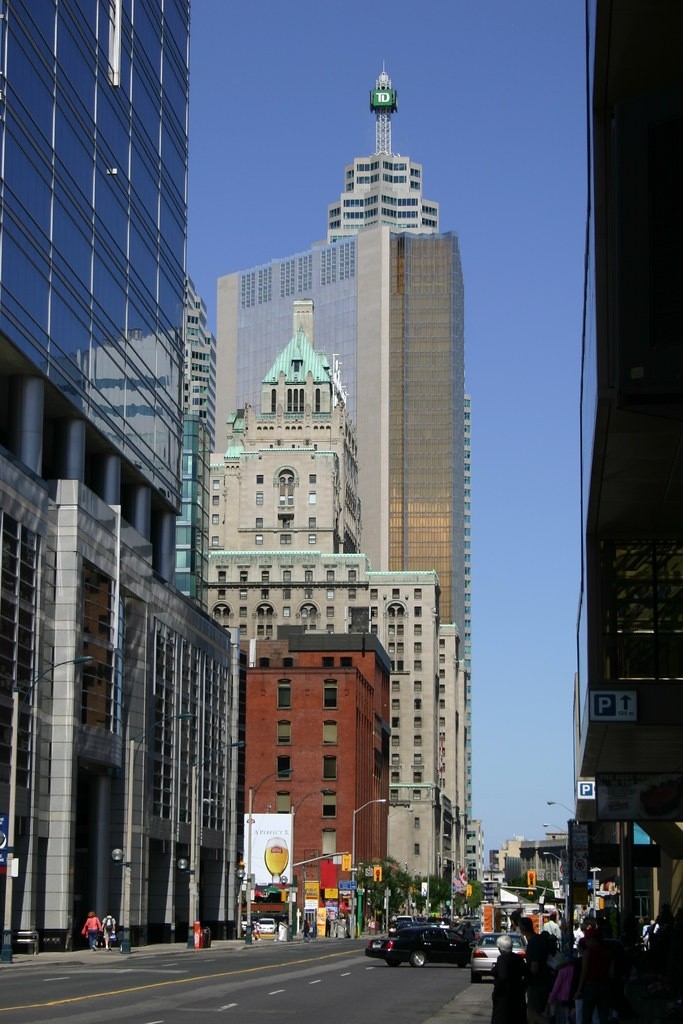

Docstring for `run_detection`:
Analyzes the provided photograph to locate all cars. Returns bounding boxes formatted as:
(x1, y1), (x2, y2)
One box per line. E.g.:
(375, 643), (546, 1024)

(469, 932), (527, 983)
(365, 915), (480, 968)
(242, 918), (277, 934)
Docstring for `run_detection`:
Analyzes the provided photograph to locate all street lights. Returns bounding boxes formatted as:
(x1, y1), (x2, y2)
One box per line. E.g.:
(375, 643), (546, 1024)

(426, 834), (449, 924)
(0, 655), (94, 964)
(177, 741), (249, 949)
(350, 799), (387, 939)
(236, 769), (294, 945)
(388, 808), (414, 935)
(280, 788), (334, 941)
(590, 868), (601, 918)
(110, 713), (199, 954)
(543, 852), (567, 920)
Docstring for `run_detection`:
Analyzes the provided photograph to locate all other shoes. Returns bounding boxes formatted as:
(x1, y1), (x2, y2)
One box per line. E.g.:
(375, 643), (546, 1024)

(91, 945), (97, 951)
(106, 947), (112, 952)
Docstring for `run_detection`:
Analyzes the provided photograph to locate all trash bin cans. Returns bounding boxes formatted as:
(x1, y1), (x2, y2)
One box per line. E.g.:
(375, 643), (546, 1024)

(203, 926), (211, 948)
(278, 922), (289, 941)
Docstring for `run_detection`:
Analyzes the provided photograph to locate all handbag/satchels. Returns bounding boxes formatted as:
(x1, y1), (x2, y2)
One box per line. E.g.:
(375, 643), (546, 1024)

(109, 933), (116, 942)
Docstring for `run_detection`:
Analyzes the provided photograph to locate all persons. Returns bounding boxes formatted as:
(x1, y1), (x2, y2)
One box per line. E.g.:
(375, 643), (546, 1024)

(251, 921), (261, 940)
(101, 911), (116, 952)
(302, 920), (312, 942)
(81, 911), (101, 952)
(490, 904), (683, 1024)
(326, 916), (331, 938)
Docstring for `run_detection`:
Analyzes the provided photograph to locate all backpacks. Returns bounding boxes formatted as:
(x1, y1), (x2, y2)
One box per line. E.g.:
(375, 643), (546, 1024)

(104, 918), (113, 931)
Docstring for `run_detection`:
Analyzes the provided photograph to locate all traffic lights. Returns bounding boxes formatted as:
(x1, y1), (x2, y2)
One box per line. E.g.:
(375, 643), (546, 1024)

(527, 870), (536, 888)
(413, 888), (416, 893)
(374, 867), (382, 882)
(528, 891), (533, 896)
(467, 885), (471, 896)
(599, 898), (604, 909)
